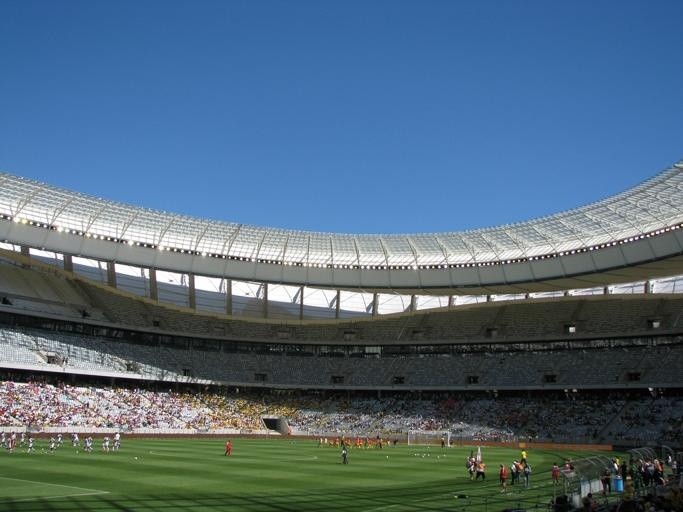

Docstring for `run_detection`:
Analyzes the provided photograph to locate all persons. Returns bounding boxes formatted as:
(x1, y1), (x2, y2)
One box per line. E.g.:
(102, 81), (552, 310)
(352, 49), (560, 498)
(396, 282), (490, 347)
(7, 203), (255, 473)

(1, 371), (683, 511)
